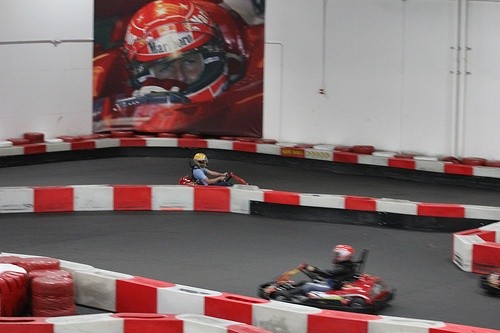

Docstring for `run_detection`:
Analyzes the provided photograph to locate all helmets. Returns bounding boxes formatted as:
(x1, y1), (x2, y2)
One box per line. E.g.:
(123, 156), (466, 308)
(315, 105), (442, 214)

(331, 243), (353, 262)
(193, 153), (209, 170)
(123, 0), (231, 107)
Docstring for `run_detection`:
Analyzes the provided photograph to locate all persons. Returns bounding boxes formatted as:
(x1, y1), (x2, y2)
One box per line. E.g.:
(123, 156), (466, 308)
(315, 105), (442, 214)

(277, 244), (356, 300)
(93, 0), (262, 134)
(192, 152), (232, 186)
